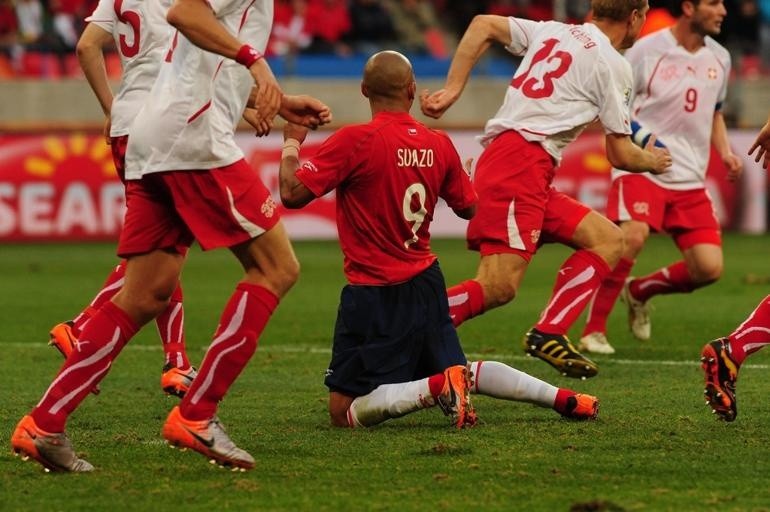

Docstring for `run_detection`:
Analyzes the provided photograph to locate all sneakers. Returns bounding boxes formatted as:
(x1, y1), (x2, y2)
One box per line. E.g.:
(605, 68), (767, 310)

(437, 364), (478, 430)
(578, 332), (615, 354)
(700, 337), (737, 422)
(162, 405), (257, 475)
(521, 326), (599, 381)
(48, 318), (102, 396)
(10, 416), (93, 473)
(620, 276), (652, 341)
(160, 363), (197, 397)
(563, 392), (600, 419)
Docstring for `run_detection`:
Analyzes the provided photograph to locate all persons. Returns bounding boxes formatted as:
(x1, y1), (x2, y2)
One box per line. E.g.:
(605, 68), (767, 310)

(578, 0), (734, 356)
(278, 50), (599, 433)
(45, 1), (199, 399)
(699, 109), (770, 421)
(419, 0), (672, 381)
(11, 1), (300, 476)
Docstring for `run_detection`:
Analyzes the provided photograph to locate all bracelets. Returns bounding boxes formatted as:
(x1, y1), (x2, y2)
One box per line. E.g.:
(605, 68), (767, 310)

(281, 137), (301, 161)
